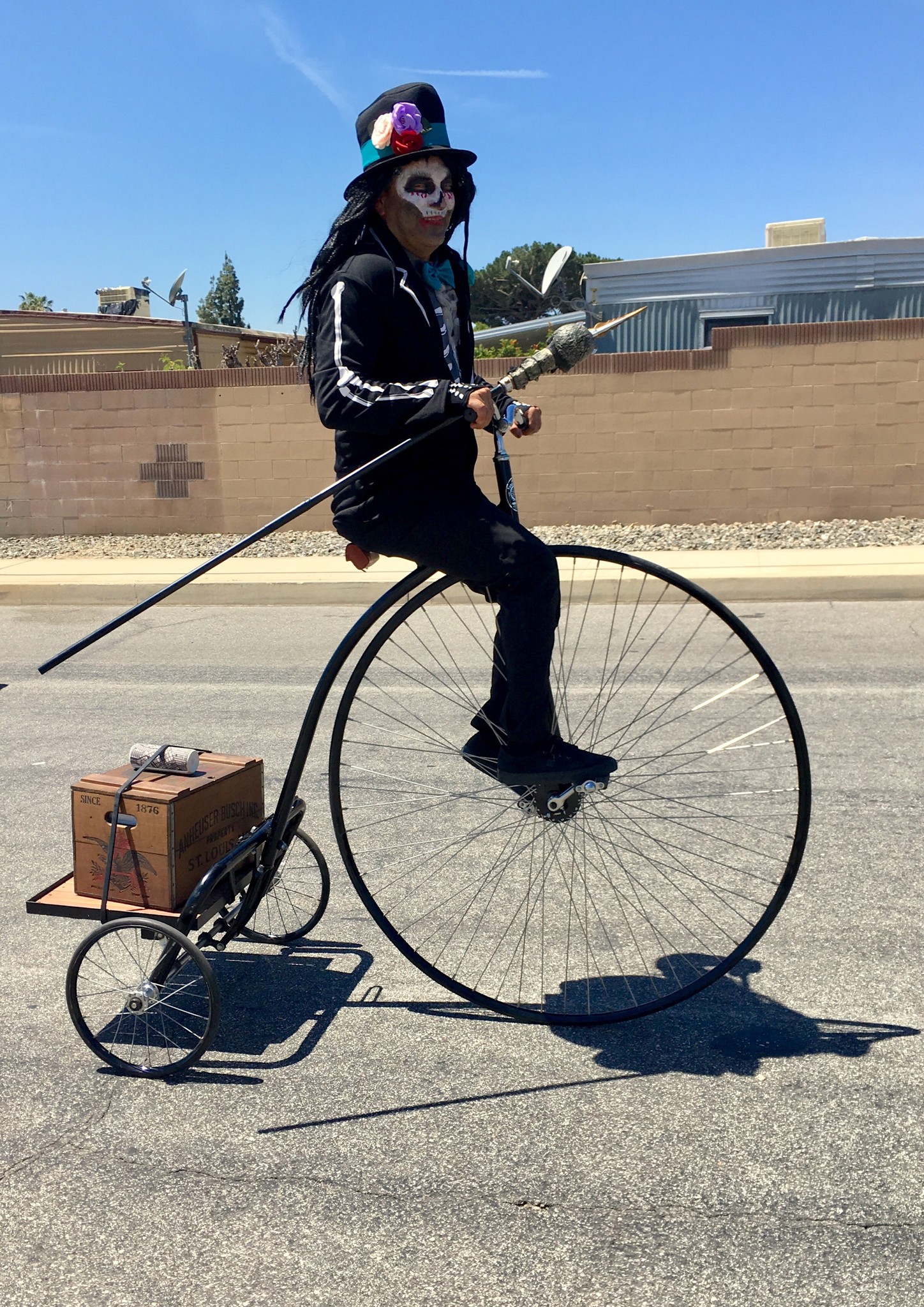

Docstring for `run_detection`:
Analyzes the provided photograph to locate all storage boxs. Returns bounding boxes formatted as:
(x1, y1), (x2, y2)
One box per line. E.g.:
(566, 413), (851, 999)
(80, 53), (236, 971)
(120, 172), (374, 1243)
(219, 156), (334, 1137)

(69, 742), (266, 912)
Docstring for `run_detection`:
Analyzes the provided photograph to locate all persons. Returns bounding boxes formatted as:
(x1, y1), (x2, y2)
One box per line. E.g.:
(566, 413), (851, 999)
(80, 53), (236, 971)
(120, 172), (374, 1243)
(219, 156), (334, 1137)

(280, 81), (617, 802)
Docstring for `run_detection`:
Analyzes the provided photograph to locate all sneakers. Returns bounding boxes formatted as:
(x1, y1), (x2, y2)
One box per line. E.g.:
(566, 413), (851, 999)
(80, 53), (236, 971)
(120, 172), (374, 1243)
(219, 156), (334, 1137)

(462, 733), (534, 801)
(496, 735), (618, 782)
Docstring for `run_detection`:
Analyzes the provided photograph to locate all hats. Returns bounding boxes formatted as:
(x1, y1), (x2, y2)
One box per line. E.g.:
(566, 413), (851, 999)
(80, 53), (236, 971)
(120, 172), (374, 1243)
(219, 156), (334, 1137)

(344, 82), (478, 202)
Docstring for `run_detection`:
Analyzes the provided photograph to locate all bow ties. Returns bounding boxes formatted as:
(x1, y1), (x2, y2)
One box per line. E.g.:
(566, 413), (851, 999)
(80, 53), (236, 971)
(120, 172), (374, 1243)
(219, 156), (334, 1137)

(423, 262), (456, 291)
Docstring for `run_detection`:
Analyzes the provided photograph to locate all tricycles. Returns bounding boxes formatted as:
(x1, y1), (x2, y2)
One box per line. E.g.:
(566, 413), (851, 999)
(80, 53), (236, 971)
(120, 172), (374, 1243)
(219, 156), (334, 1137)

(25, 382), (814, 1079)
